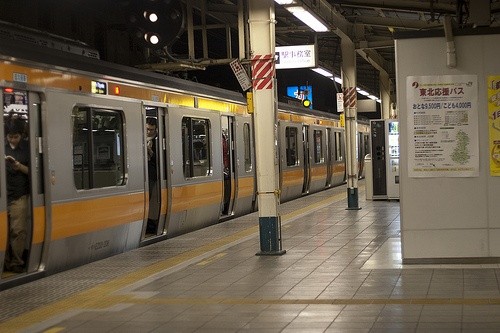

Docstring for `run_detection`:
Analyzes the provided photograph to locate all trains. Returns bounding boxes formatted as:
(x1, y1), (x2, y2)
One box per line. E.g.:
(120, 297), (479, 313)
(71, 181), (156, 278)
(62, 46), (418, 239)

(1, 16), (373, 295)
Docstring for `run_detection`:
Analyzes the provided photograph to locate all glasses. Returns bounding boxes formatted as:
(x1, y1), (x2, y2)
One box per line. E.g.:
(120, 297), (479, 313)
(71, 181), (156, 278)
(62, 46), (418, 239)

(147, 128), (156, 131)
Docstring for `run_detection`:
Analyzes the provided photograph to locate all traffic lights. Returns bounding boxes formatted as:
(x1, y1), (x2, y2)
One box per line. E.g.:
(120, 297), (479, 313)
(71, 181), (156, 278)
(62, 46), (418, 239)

(302, 98), (311, 107)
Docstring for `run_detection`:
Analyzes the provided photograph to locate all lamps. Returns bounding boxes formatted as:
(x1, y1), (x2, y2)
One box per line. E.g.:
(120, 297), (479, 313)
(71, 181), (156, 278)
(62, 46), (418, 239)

(311, 66), (382, 104)
(284, 3), (331, 32)
(274, 0), (300, 5)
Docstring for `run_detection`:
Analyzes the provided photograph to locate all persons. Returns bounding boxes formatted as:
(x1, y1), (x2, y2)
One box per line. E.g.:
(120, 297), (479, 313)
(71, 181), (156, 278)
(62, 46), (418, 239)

(3, 116), (33, 273)
(220, 133), (230, 217)
(144, 117), (159, 201)
